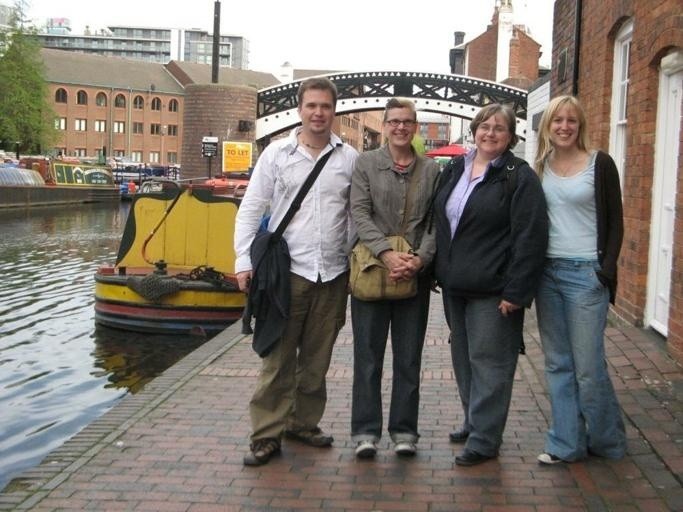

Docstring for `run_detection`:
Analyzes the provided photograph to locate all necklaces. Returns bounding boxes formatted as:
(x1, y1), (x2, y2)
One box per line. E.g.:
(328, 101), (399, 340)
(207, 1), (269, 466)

(300, 135), (330, 149)
(553, 150), (579, 177)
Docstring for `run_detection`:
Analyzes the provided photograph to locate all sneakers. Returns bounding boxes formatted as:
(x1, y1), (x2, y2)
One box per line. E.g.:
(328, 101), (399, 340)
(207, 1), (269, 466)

(394, 441), (417, 456)
(456, 448), (491, 465)
(354, 439), (377, 458)
(537, 453), (563, 464)
(449, 426), (469, 441)
(285, 426), (333, 446)
(244, 438), (281, 465)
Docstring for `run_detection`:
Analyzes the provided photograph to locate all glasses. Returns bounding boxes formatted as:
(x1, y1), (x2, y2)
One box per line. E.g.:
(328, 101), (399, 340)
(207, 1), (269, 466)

(386, 119), (416, 127)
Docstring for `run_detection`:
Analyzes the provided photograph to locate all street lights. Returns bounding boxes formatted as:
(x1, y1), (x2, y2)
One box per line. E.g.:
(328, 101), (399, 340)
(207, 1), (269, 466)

(15, 141), (21, 160)
(161, 131), (167, 167)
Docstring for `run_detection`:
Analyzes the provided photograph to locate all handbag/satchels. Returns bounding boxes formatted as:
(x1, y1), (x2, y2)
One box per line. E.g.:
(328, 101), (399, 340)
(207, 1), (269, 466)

(246, 235), (281, 321)
(348, 236), (419, 302)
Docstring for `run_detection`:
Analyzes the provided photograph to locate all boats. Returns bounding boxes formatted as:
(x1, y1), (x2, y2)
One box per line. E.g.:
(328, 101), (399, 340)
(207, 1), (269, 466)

(91, 173), (252, 341)
(0, 155), (124, 209)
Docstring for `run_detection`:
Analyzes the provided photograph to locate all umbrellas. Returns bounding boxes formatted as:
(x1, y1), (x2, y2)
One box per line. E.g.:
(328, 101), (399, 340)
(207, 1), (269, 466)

(425, 143), (467, 157)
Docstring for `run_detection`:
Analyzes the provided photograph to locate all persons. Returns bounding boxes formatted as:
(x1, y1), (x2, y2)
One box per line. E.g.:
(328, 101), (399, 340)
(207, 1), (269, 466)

(347, 99), (442, 458)
(233, 78), (361, 466)
(428, 104), (549, 465)
(530, 92), (628, 463)
